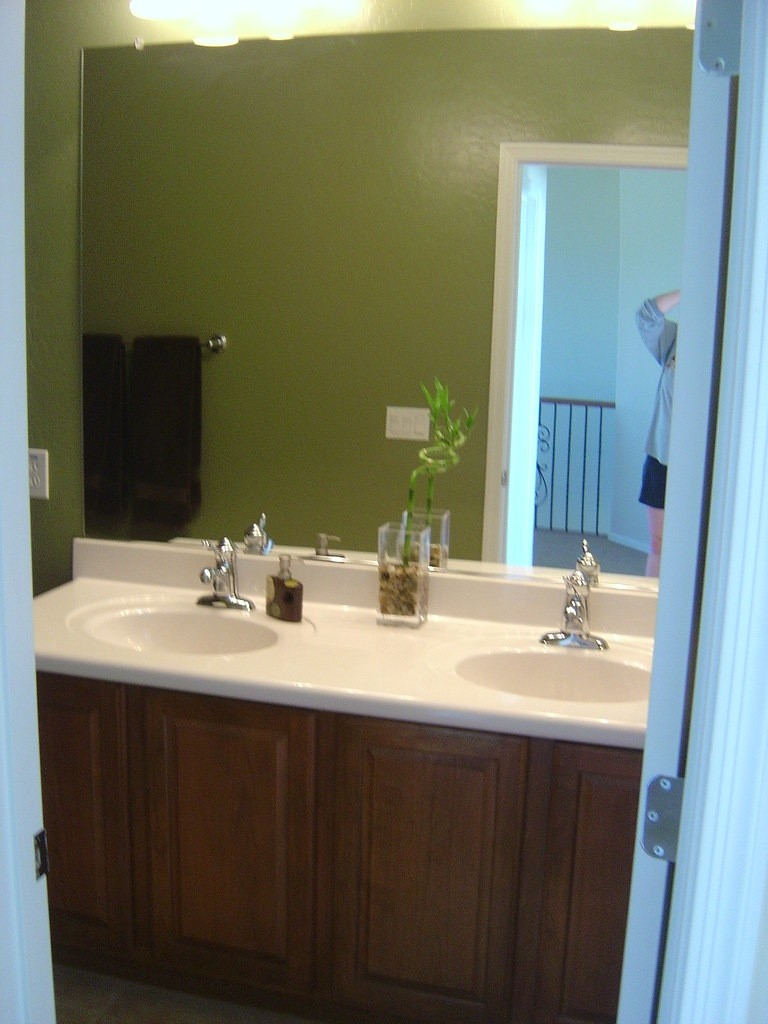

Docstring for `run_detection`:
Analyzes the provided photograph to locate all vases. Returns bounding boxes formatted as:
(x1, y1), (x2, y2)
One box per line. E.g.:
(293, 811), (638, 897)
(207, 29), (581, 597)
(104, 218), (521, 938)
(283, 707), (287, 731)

(374, 520), (433, 629)
(401, 506), (451, 572)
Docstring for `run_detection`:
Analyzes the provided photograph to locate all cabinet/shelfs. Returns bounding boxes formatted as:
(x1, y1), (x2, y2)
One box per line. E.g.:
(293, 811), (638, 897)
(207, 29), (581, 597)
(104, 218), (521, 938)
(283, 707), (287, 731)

(34, 574), (643, 1024)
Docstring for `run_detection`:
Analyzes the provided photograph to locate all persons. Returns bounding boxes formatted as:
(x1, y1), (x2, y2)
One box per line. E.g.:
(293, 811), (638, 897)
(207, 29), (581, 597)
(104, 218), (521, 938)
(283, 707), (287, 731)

(635, 289), (680, 576)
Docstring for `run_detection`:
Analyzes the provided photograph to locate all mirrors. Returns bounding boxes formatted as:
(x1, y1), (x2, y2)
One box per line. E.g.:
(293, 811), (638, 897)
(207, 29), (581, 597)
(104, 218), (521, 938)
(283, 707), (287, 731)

(78, 22), (695, 595)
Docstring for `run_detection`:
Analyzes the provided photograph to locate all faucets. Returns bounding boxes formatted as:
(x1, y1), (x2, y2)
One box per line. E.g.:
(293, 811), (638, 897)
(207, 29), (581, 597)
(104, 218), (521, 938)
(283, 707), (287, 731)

(576, 537), (599, 587)
(196, 536), (253, 610)
(241, 511), (274, 556)
(541, 574), (608, 651)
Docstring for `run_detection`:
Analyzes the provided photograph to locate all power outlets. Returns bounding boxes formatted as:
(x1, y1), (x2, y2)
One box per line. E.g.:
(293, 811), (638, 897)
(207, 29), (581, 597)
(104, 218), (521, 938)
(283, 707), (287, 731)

(29, 448), (50, 499)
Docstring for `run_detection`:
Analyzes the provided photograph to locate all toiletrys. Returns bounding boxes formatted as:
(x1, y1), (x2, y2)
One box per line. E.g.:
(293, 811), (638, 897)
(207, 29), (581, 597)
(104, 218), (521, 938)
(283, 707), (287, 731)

(265, 553), (304, 623)
(308, 534), (345, 557)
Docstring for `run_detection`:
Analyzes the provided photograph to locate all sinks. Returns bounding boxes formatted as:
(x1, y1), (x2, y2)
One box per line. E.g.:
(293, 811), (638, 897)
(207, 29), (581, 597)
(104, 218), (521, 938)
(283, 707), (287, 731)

(66, 594), (316, 666)
(431, 632), (654, 711)
